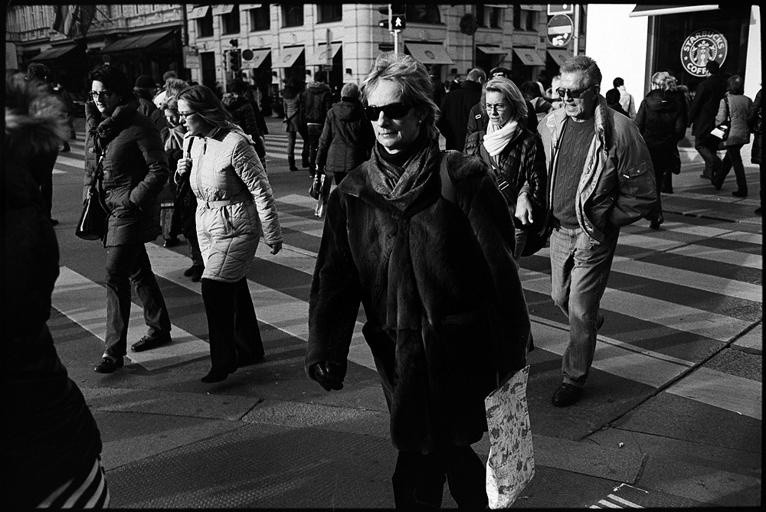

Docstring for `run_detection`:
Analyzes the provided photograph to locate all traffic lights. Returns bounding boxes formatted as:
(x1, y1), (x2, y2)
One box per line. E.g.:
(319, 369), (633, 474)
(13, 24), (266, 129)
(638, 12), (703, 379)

(378, 4), (391, 31)
(391, 15), (405, 29)
(221, 51), (229, 72)
(228, 39), (240, 48)
(231, 49), (241, 71)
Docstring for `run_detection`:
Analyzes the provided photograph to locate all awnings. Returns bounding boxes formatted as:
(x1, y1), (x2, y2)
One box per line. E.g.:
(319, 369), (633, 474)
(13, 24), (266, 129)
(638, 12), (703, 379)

(307, 43), (341, 66)
(241, 50), (271, 69)
(271, 46), (305, 68)
(515, 48), (547, 65)
(404, 44), (454, 65)
(548, 49), (570, 66)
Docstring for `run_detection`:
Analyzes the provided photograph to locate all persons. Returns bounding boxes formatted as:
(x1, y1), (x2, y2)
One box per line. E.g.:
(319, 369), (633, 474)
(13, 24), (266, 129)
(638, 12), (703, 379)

(224, 80), (269, 170)
(430, 67), (561, 153)
(81, 62), (173, 375)
(29, 65), (205, 282)
(171, 84), (282, 386)
(2, 68), (111, 510)
(460, 71), (548, 357)
(513, 55), (660, 408)
(300, 51), (536, 511)
(607, 60), (764, 232)
(283, 70), (375, 186)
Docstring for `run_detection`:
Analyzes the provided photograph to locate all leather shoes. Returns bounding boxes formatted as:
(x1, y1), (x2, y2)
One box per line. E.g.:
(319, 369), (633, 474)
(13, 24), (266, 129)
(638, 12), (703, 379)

(201, 369), (228, 383)
(92, 359), (122, 373)
(130, 334), (170, 352)
(163, 238), (181, 247)
(649, 212), (664, 230)
(551, 383), (580, 407)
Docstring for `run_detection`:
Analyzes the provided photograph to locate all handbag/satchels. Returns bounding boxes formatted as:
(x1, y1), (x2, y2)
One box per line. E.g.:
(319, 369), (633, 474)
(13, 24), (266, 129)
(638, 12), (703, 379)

(74, 187), (107, 241)
(485, 364), (537, 509)
(174, 138), (197, 219)
(708, 97), (731, 141)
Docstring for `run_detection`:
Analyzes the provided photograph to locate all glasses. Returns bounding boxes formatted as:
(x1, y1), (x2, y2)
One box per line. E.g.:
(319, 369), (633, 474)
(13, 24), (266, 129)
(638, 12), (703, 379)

(175, 110), (196, 119)
(87, 90), (112, 97)
(481, 103), (508, 111)
(555, 86), (590, 98)
(363, 101), (418, 121)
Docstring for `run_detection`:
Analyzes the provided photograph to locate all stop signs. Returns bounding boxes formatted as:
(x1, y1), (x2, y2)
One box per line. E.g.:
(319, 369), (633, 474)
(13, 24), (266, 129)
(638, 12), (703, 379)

(545, 15), (573, 47)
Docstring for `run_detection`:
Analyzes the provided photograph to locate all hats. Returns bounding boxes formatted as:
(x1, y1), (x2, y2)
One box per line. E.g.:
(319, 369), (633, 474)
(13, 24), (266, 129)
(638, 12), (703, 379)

(340, 84), (358, 98)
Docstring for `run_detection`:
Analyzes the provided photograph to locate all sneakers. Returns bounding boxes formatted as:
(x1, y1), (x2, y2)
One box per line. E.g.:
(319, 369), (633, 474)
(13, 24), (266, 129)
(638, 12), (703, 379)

(184, 265), (195, 277)
(191, 267), (202, 282)
